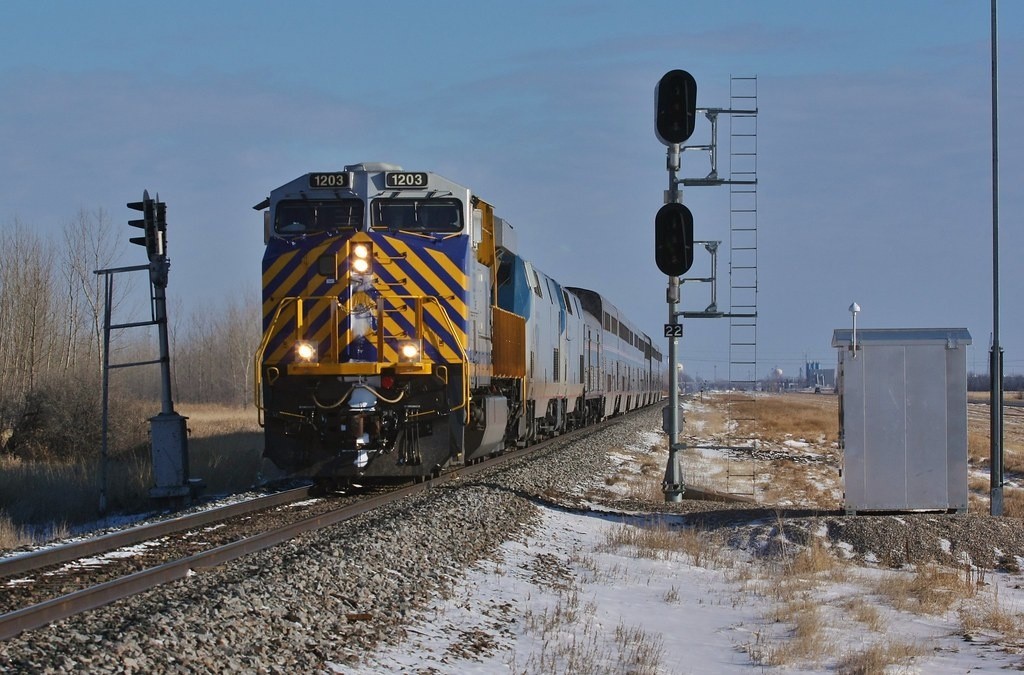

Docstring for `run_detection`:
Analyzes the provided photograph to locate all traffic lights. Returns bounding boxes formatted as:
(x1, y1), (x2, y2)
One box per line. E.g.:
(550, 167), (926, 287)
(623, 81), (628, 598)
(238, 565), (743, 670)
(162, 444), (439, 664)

(126, 189), (152, 259)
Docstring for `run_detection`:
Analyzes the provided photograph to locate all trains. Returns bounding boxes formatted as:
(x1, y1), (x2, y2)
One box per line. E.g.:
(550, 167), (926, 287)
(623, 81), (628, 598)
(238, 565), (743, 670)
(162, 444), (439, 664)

(254, 163), (665, 489)
